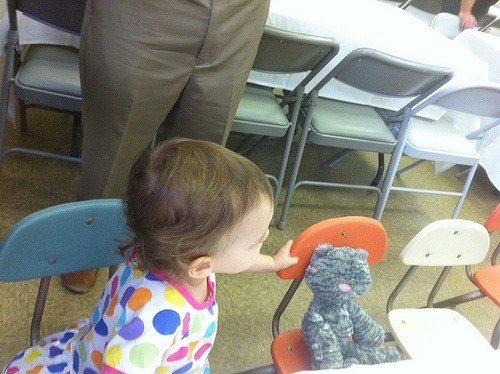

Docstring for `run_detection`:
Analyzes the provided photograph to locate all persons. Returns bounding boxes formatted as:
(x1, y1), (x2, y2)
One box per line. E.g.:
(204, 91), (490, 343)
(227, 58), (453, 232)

(440, 0), (498, 32)
(61, 1), (270, 293)
(2, 137), (300, 374)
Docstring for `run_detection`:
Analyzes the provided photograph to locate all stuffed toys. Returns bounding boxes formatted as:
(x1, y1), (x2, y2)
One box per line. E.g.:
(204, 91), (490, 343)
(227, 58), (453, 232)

(300, 242), (400, 370)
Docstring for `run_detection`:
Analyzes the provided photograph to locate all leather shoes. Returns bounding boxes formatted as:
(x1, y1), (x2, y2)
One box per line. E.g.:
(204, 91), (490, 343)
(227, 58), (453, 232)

(62, 267), (100, 292)
(108, 264), (118, 280)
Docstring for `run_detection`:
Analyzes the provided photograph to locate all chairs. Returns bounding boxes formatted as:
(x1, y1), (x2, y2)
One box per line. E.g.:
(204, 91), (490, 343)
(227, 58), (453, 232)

(387, 200), (500, 359)
(270, 216), (388, 373)
(0, 0), (500, 230)
(0, 198), (137, 346)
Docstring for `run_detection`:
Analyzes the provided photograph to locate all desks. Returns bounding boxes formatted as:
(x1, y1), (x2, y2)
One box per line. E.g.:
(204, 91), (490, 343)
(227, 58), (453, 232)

(444, 28), (500, 193)
(1, 0), (488, 174)
